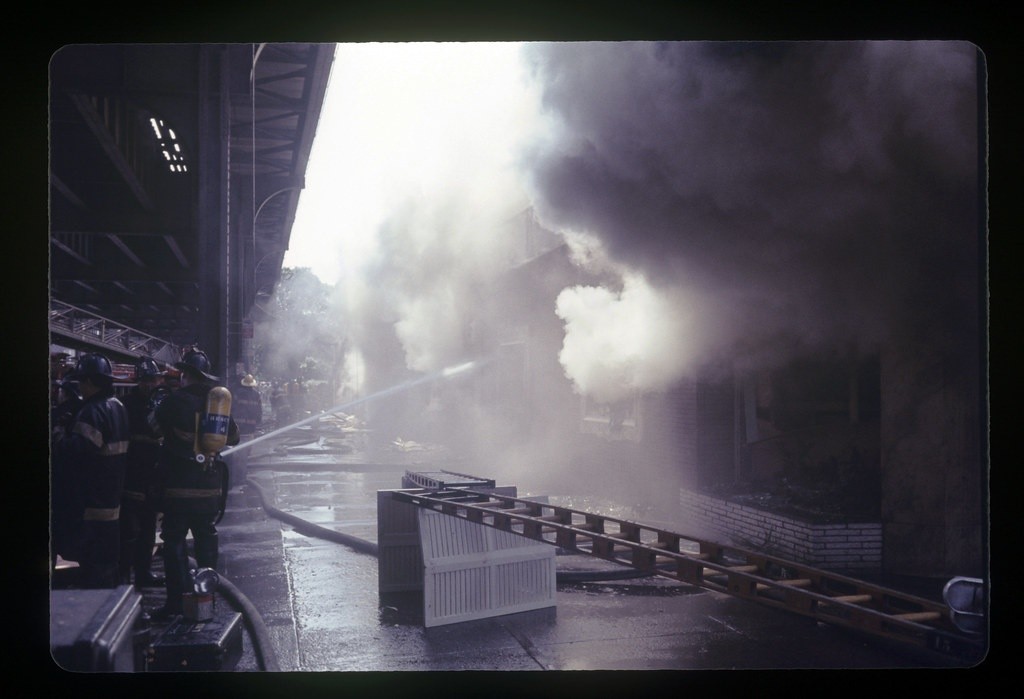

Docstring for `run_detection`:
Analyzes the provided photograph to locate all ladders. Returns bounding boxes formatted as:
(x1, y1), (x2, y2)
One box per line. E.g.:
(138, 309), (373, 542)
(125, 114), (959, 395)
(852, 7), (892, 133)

(404, 467), (496, 491)
(389, 487), (986, 657)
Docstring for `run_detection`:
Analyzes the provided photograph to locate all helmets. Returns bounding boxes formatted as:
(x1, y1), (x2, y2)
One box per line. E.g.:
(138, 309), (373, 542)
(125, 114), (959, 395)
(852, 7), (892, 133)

(173, 351), (223, 381)
(241, 374), (256, 386)
(70, 352), (120, 380)
(149, 386), (173, 410)
(53, 371), (83, 401)
(130, 356), (169, 378)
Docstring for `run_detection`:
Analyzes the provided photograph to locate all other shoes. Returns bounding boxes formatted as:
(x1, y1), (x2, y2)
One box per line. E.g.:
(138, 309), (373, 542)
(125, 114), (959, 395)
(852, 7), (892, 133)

(135, 572), (168, 587)
(144, 605), (195, 624)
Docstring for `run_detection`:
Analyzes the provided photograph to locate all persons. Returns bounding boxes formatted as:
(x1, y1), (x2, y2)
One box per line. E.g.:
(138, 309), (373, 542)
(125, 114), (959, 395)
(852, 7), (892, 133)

(270, 373), (311, 435)
(51, 341), (265, 625)
